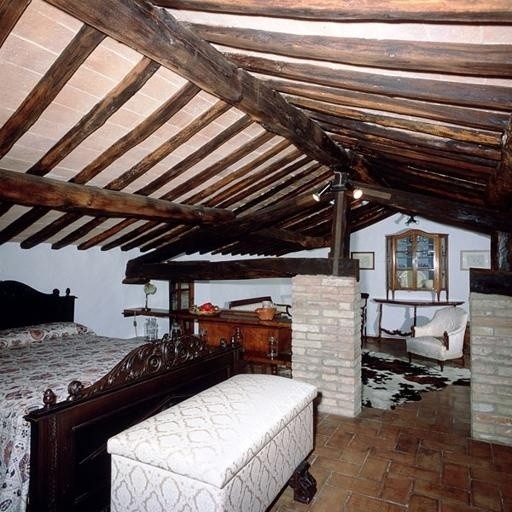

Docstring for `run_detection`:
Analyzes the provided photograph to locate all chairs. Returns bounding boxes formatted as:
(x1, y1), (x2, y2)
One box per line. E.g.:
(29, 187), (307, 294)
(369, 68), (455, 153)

(406, 306), (468, 372)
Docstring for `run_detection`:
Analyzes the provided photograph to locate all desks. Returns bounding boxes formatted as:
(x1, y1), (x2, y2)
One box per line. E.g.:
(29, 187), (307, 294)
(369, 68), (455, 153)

(374, 298), (465, 342)
(124, 311), (292, 371)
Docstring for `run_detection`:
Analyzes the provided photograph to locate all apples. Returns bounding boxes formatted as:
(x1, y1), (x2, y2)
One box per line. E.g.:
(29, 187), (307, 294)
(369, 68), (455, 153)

(200, 304), (214, 312)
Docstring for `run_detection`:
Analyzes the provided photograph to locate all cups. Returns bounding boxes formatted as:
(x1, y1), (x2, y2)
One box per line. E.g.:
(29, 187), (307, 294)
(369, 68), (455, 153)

(266, 336), (279, 358)
(261, 301), (274, 310)
(144, 318), (158, 341)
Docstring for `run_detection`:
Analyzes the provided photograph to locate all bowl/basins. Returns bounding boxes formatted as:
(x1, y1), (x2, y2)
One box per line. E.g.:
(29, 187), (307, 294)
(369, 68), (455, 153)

(424, 279), (433, 289)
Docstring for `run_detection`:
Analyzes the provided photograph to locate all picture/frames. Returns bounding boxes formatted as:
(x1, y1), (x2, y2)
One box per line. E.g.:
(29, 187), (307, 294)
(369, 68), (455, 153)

(351, 252), (374, 271)
(460, 250), (490, 270)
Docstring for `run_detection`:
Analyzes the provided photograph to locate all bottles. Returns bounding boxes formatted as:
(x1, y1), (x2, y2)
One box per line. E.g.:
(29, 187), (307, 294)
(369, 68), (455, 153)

(231, 327), (245, 353)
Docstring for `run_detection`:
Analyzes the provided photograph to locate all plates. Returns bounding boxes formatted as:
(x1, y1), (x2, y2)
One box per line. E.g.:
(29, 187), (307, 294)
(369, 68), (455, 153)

(399, 271), (425, 289)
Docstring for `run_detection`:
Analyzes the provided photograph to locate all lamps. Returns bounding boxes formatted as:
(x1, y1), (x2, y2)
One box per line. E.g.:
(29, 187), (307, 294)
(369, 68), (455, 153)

(312, 178), (369, 210)
(405, 216), (420, 227)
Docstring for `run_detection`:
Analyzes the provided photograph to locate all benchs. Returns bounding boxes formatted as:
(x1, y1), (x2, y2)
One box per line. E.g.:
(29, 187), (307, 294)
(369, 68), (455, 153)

(107, 373), (319, 512)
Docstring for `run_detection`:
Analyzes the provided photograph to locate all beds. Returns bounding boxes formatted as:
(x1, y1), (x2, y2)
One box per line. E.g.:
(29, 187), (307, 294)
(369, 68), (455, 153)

(0, 279), (240, 511)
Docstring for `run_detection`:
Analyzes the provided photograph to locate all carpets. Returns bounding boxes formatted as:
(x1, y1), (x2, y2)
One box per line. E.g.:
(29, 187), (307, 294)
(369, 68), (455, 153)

(362, 352), (471, 411)
